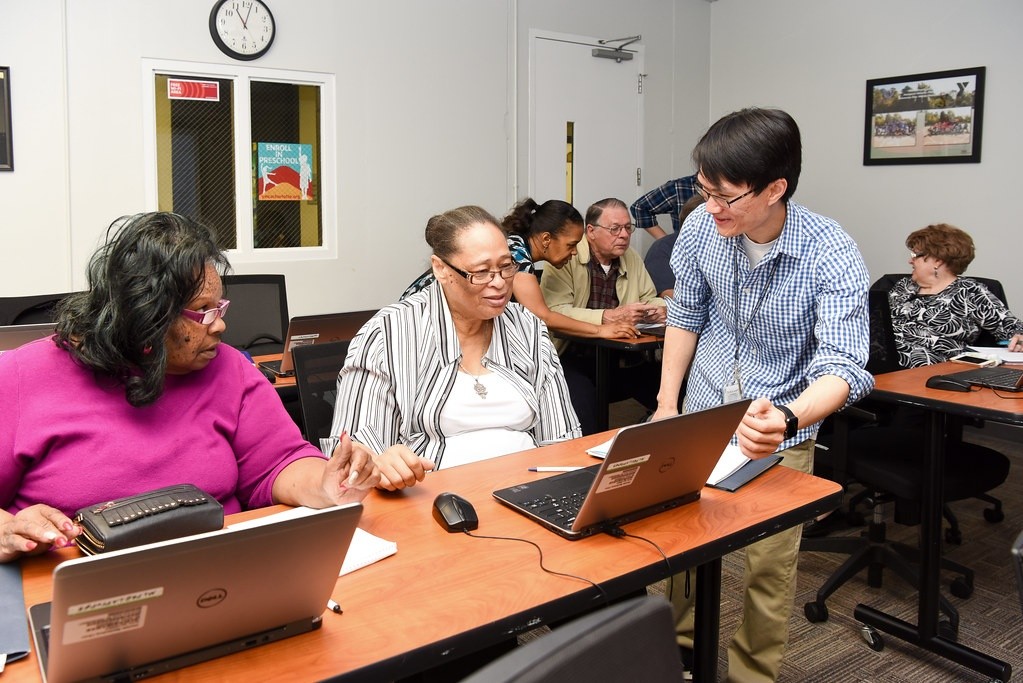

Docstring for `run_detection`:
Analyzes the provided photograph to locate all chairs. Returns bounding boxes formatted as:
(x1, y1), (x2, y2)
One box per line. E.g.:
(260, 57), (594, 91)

(217, 275), (292, 355)
(460, 595), (689, 683)
(803, 274), (1011, 651)
(293, 340), (354, 453)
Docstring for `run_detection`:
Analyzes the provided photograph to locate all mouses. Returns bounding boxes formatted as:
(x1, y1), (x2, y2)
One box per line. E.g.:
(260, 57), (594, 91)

(258, 367), (276, 383)
(433, 492), (478, 530)
(925, 374), (971, 392)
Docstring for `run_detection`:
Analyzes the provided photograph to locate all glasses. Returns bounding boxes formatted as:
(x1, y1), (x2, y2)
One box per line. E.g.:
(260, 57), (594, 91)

(594, 224), (636, 236)
(910, 252), (926, 259)
(182, 298), (229, 325)
(434, 254), (519, 287)
(694, 182), (753, 210)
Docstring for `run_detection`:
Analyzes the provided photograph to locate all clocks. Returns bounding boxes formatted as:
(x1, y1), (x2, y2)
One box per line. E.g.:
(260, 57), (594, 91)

(209, 0), (276, 62)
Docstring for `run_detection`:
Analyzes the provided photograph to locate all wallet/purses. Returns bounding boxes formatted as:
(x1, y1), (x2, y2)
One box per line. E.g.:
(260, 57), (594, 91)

(72, 483), (226, 559)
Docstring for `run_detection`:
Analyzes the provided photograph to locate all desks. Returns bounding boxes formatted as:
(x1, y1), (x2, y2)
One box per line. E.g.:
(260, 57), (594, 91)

(552, 325), (666, 434)
(251, 353), (350, 401)
(865, 359), (1023, 683)
(0, 424), (844, 683)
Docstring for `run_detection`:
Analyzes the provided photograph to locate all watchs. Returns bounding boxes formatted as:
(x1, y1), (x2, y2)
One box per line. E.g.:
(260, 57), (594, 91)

(776, 405), (799, 441)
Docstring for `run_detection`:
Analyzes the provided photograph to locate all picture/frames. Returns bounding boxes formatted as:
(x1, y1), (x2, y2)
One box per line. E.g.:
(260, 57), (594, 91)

(863, 66), (986, 166)
(0, 66), (15, 172)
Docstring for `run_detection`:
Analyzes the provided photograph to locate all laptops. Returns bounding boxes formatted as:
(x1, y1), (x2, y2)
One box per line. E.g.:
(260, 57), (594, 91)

(492, 396), (754, 541)
(258, 310), (379, 378)
(28, 502), (365, 683)
(942, 366), (1023, 392)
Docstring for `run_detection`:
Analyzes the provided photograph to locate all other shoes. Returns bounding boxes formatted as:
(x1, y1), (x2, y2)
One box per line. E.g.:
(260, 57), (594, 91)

(801, 508), (847, 537)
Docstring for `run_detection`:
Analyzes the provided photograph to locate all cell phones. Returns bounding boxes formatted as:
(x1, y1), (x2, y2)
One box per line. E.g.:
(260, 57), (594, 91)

(952, 356), (996, 368)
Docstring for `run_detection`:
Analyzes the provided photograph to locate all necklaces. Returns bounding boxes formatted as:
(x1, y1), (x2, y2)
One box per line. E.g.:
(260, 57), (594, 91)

(459, 318), (488, 399)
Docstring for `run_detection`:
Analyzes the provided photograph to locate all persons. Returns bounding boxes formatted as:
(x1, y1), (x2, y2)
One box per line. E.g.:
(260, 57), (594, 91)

(397, 198), (642, 340)
(869, 224), (1023, 370)
(644, 195), (706, 300)
(630, 173), (701, 241)
(319, 206), (584, 491)
(0, 211), (381, 559)
(540, 197), (688, 434)
(649, 106), (875, 683)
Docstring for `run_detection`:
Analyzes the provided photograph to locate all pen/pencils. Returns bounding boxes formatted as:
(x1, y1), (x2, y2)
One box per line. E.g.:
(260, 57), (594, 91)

(996, 340), (1023, 345)
(528, 466), (585, 472)
(326, 599), (340, 612)
